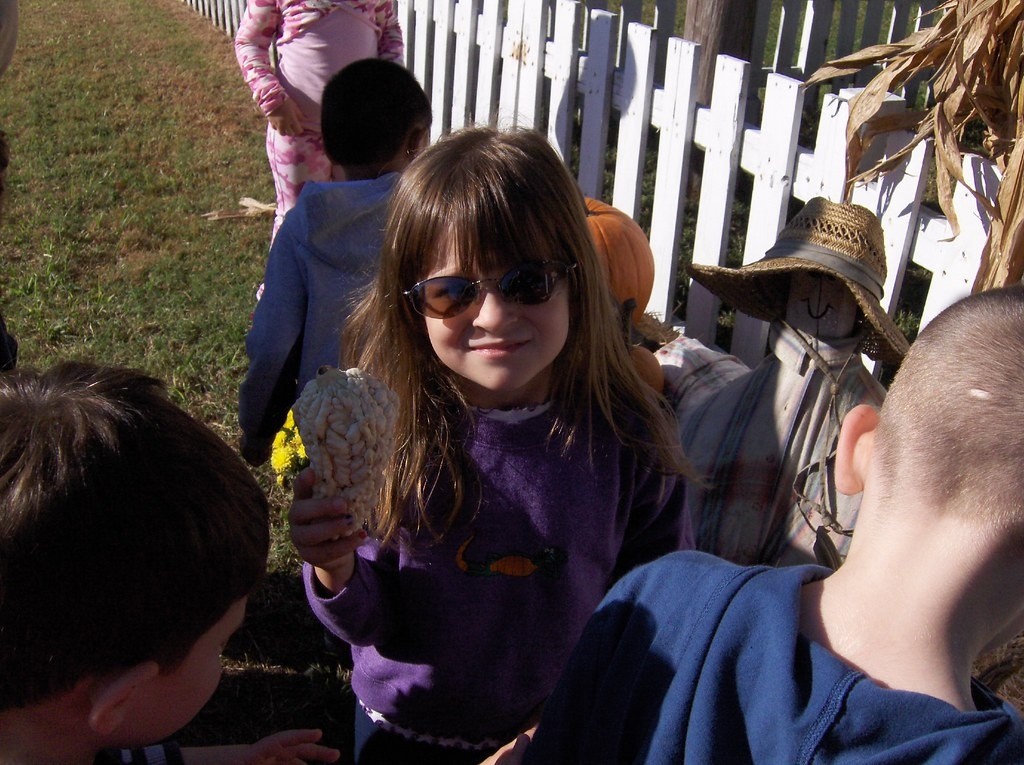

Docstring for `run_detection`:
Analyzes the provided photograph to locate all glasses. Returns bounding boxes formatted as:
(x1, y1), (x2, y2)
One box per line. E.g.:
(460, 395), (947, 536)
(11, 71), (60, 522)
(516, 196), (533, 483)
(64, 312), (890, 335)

(402, 259), (576, 319)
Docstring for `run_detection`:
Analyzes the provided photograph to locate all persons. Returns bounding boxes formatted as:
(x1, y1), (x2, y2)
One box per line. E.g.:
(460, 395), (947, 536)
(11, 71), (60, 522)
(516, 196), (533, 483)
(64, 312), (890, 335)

(0, 0), (709, 765)
(501, 283), (1024, 765)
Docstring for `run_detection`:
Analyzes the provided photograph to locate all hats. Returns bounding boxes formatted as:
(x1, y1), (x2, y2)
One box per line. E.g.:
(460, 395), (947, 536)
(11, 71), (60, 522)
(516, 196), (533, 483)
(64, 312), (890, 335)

(686, 196), (911, 365)
